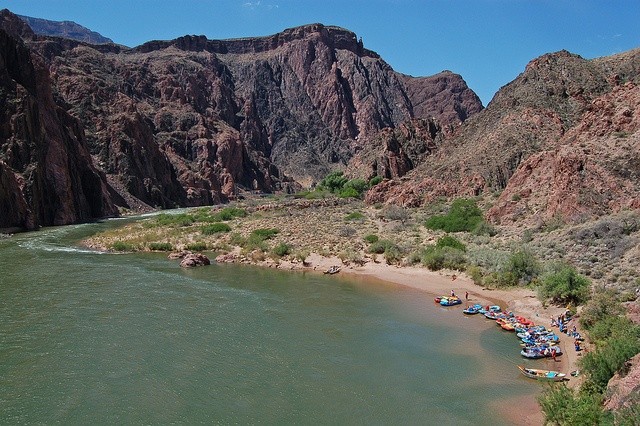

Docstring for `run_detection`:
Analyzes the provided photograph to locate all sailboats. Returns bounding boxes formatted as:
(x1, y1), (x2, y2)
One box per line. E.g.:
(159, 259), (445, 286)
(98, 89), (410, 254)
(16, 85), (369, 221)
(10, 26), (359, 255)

(463, 307), (478, 313)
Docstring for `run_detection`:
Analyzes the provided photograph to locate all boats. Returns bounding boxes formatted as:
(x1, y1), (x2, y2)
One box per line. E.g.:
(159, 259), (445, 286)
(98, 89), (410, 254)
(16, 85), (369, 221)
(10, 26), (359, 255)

(479, 305), (500, 313)
(440, 295), (462, 305)
(484, 310), (503, 319)
(516, 364), (566, 380)
(433, 295), (442, 302)
(323, 264), (342, 273)
(517, 325), (554, 338)
(501, 320), (535, 329)
(496, 315), (525, 325)
(520, 341), (561, 357)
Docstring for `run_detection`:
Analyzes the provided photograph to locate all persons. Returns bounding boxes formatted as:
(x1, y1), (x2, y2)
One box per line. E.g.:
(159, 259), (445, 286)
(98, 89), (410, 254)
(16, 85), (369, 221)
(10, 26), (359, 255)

(451, 290), (454, 296)
(485, 305), (489, 311)
(465, 292), (468, 300)
(551, 348), (558, 361)
(550, 313), (584, 351)
